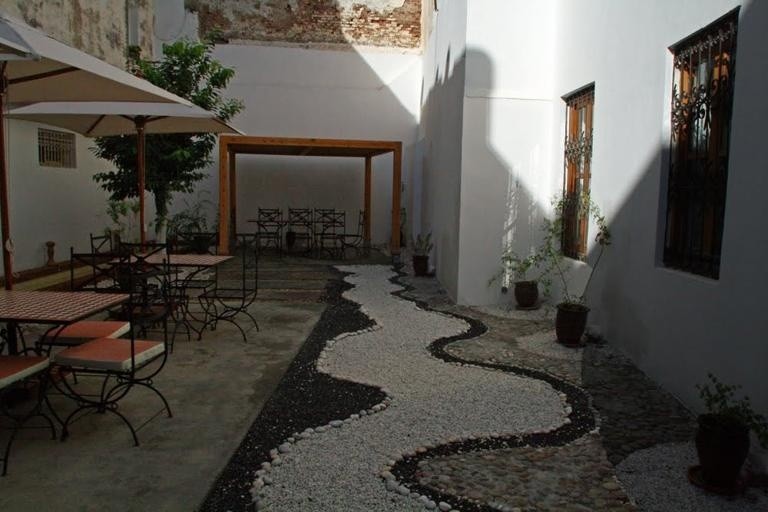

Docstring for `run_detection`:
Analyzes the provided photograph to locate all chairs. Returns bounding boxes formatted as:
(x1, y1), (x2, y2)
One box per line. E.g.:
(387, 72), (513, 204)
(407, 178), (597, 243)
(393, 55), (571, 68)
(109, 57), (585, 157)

(0, 353), (55, 475)
(67, 230), (261, 353)
(55, 254), (174, 446)
(39, 246), (133, 401)
(250, 205), (368, 259)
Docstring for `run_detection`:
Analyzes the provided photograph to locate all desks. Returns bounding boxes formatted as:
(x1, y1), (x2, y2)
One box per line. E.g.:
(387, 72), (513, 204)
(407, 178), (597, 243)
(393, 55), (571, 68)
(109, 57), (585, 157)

(0, 290), (131, 434)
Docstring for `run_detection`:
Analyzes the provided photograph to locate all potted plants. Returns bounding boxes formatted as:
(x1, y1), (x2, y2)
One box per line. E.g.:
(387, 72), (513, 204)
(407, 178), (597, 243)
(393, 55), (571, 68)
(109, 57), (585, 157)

(487, 238), (562, 309)
(537, 183), (608, 343)
(410, 232), (432, 274)
(690, 367), (761, 491)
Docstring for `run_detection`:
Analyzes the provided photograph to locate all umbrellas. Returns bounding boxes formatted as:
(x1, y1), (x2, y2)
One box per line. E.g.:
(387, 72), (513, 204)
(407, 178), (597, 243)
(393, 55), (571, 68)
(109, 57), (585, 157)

(4, 102), (246, 254)
(0, 16), (194, 290)
